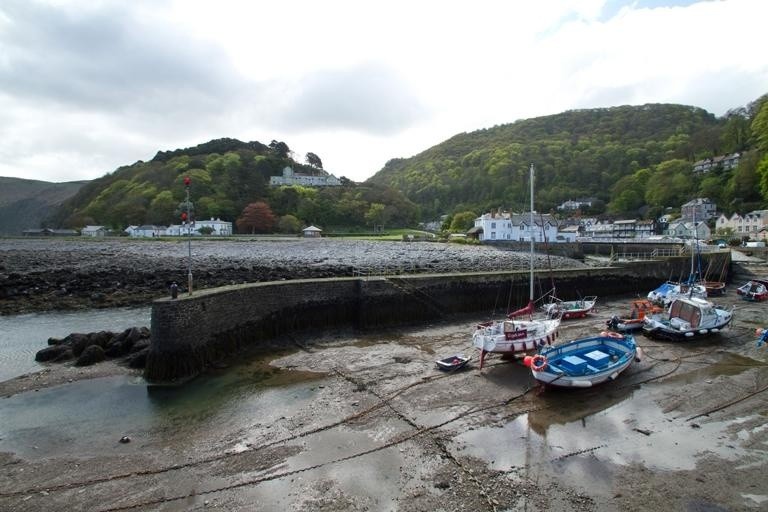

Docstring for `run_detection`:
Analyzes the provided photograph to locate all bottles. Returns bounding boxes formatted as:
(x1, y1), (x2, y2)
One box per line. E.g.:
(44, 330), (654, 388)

(171, 281), (178, 299)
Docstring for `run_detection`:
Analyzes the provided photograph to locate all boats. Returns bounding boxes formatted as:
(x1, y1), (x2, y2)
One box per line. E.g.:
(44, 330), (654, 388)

(531, 332), (643, 396)
(526, 385), (640, 435)
(435, 354), (470, 371)
(611, 202), (737, 345)
(737, 278), (767, 302)
(540, 289), (601, 320)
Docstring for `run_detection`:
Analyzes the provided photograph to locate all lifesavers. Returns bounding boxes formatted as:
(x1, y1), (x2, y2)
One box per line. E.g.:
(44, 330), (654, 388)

(608, 331), (624, 340)
(532, 355), (547, 371)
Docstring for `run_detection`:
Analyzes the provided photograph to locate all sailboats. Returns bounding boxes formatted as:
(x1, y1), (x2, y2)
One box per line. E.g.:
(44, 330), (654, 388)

(466, 163), (565, 363)
